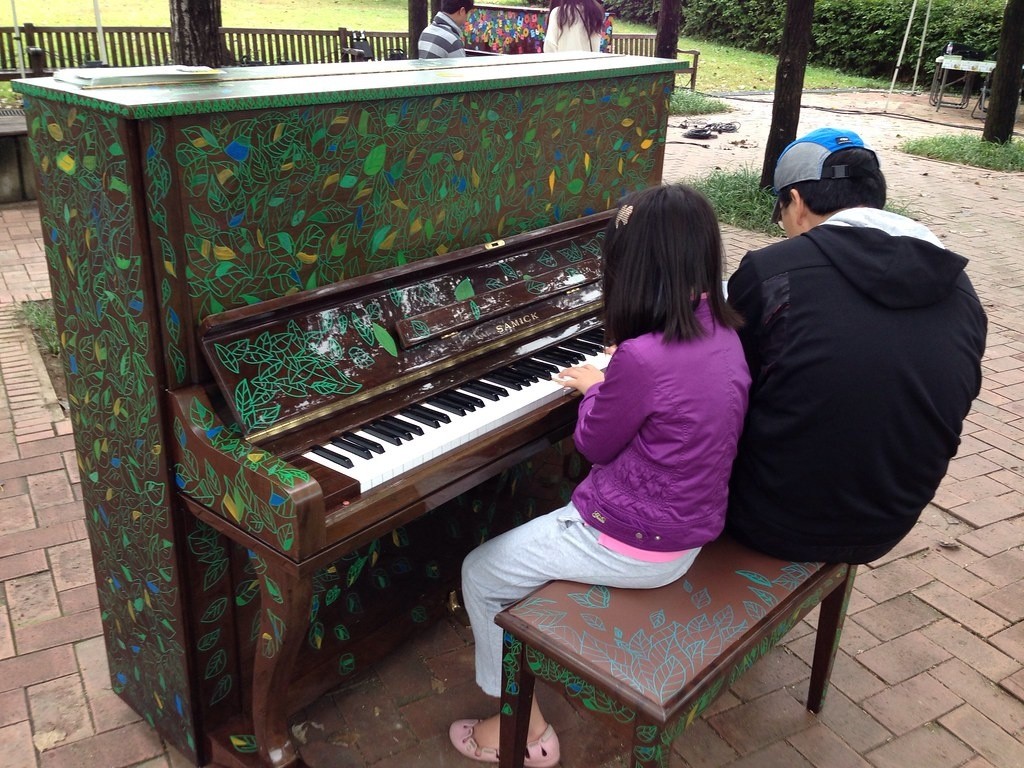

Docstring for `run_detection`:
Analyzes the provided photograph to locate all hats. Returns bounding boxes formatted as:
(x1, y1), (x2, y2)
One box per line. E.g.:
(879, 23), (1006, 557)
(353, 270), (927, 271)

(770, 128), (880, 224)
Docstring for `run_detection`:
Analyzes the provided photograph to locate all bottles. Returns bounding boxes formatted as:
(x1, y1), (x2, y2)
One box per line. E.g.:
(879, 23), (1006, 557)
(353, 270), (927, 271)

(945, 42), (953, 54)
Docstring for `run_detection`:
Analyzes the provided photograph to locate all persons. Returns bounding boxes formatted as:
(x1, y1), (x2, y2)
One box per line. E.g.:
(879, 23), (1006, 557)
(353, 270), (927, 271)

(417, 0), (473, 59)
(541, 0), (605, 53)
(723, 126), (988, 566)
(448, 182), (751, 768)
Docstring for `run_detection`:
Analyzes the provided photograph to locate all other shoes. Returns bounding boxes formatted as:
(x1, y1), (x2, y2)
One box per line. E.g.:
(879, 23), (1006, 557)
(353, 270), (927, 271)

(450, 719), (560, 768)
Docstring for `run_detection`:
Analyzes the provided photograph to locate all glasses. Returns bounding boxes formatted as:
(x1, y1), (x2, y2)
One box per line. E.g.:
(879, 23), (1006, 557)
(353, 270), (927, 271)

(776, 201), (795, 230)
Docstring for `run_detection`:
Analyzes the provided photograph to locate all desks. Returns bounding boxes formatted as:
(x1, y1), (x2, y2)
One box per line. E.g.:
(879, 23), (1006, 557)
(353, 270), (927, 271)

(935, 59), (1024, 112)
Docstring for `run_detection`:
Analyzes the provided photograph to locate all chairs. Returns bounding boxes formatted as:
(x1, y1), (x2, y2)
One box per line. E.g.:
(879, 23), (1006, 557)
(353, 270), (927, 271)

(971, 68), (996, 124)
(929, 42), (982, 109)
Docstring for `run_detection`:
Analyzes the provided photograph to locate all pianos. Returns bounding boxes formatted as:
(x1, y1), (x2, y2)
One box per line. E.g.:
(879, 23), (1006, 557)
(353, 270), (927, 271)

(7, 48), (692, 762)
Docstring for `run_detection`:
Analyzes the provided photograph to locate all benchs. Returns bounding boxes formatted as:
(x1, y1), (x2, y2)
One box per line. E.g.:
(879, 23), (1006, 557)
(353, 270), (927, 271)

(0, 19), (702, 92)
(492, 540), (860, 768)
